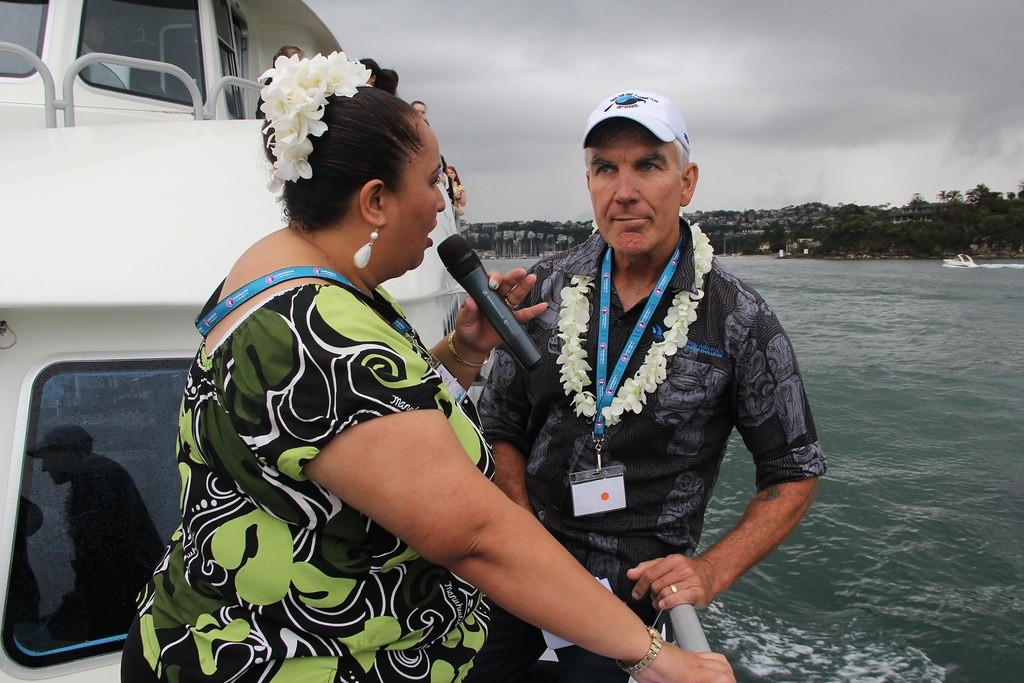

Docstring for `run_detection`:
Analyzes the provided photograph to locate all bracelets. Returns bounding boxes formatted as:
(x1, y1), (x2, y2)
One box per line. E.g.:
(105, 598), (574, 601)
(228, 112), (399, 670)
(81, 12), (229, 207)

(448, 329), (491, 367)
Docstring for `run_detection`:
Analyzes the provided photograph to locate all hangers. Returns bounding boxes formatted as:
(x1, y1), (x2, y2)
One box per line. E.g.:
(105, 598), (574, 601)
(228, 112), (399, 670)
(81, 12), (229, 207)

(128, 26), (159, 51)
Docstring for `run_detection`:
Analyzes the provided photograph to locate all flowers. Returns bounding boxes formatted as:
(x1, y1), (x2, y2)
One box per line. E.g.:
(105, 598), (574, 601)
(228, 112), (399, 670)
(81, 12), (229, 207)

(255, 49), (373, 195)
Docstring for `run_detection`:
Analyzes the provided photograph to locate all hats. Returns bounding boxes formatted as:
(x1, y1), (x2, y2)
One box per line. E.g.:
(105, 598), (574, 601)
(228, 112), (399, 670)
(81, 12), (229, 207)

(582, 92), (690, 153)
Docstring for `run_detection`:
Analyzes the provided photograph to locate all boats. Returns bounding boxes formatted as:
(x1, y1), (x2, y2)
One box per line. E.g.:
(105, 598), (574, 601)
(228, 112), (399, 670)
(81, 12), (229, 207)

(943, 254), (976, 268)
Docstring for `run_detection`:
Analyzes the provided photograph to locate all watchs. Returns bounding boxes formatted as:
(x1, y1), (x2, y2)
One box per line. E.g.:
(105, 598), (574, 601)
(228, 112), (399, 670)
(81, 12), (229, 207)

(615, 626), (663, 674)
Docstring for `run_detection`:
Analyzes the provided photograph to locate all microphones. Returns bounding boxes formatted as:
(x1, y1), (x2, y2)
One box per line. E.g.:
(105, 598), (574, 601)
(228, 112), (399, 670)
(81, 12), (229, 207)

(437, 234), (544, 374)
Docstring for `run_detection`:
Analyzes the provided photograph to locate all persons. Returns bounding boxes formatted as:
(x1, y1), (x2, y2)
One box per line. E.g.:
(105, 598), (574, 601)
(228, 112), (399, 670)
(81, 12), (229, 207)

(8, 422), (165, 655)
(464, 92), (828, 683)
(256, 45), (467, 216)
(121, 51), (733, 683)
(79, 18), (127, 90)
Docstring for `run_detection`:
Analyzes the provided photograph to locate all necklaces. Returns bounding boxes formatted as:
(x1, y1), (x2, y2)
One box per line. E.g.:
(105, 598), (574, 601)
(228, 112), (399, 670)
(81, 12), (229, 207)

(559, 217), (715, 428)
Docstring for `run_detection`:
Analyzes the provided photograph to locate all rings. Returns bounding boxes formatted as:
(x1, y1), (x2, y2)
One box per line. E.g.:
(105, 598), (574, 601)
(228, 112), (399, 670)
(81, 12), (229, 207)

(506, 294), (519, 309)
(670, 584), (678, 593)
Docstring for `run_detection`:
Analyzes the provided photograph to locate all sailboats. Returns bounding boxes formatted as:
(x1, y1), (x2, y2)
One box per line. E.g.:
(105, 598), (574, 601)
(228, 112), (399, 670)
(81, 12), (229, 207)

(721, 237), (744, 257)
(471, 227), (574, 261)
